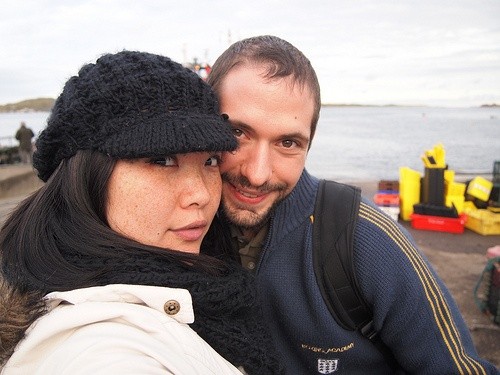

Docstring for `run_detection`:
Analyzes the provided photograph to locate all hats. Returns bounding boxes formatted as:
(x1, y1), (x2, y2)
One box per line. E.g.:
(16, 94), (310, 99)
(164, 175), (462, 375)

(33, 50), (239, 181)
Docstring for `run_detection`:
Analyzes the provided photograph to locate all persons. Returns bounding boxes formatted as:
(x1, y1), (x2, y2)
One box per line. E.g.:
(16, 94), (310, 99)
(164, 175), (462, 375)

(204, 35), (500, 375)
(16, 122), (34, 164)
(0, 51), (285, 375)
